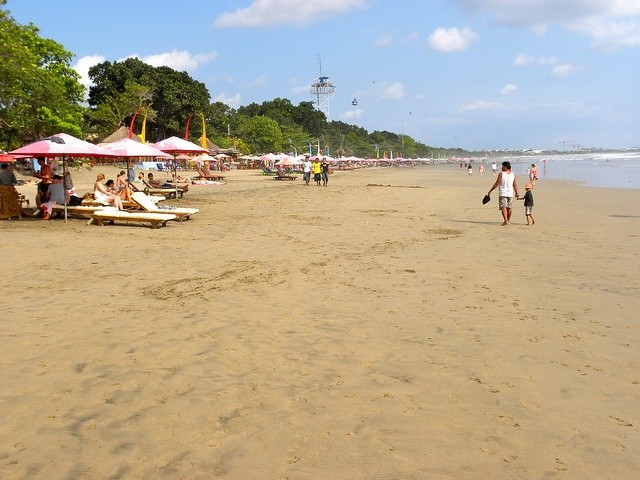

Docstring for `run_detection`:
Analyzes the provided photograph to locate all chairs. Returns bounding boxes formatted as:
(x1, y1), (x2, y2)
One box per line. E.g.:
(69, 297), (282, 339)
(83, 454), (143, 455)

(40, 204), (119, 219)
(90, 211), (177, 229)
(273, 170), (298, 180)
(142, 178), (184, 198)
(130, 190), (200, 222)
(194, 167), (219, 181)
(157, 163), (167, 172)
(81, 199), (139, 210)
(261, 165), (278, 176)
(161, 182), (189, 193)
(201, 168), (226, 180)
(130, 179), (177, 199)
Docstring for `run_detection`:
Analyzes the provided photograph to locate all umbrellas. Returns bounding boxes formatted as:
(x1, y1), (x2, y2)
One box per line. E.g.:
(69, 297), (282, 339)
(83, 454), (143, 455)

(238, 153), (305, 173)
(101, 137), (174, 187)
(149, 135), (210, 179)
(305, 153), (330, 162)
(8, 133), (118, 222)
(330, 155), (468, 170)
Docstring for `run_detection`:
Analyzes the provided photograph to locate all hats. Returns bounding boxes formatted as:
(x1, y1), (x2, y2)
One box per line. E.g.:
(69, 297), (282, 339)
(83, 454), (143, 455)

(51, 175), (62, 179)
(128, 168), (135, 182)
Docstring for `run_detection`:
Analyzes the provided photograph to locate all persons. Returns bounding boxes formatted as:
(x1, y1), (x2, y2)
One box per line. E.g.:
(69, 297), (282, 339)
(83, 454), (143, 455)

(320, 158), (330, 187)
(41, 175), (64, 219)
(158, 153), (232, 161)
(64, 186), (78, 206)
(0, 164), (25, 221)
(491, 162), (497, 174)
(117, 171), (133, 193)
(192, 178), (227, 185)
(516, 184), (535, 225)
(148, 173), (176, 189)
(303, 156), (313, 185)
(94, 173), (124, 211)
(479, 164), (484, 175)
(32, 156), (53, 216)
(48, 156), (68, 175)
(106, 180), (135, 203)
(488, 162), (519, 225)
(312, 158), (323, 185)
(468, 163), (472, 175)
(529, 164), (537, 189)
(138, 172), (154, 189)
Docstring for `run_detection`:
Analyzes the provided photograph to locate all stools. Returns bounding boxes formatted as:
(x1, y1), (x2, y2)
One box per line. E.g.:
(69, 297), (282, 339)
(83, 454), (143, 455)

(16, 199), (29, 209)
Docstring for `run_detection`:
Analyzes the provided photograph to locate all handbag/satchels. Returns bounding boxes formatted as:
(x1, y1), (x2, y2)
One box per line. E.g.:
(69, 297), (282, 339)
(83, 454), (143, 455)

(63, 161), (74, 189)
(303, 174), (306, 180)
